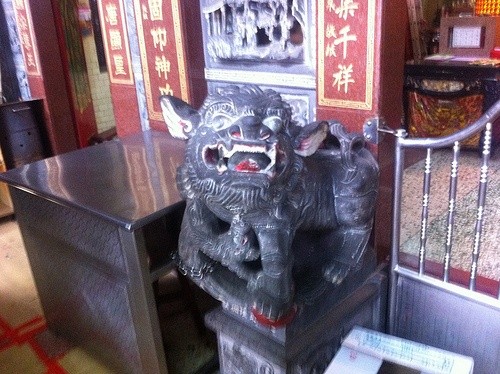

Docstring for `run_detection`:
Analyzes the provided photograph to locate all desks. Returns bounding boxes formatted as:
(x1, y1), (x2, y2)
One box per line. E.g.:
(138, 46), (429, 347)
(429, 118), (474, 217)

(402, 57), (497, 151)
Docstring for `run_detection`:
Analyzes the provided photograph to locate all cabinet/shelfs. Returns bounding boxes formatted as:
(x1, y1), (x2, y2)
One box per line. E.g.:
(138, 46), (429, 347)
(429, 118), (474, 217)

(0, 130), (225, 373)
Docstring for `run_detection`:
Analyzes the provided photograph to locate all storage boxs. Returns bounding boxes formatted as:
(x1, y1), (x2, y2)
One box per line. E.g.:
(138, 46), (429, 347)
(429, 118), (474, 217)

(318, 325), (476, 374)
